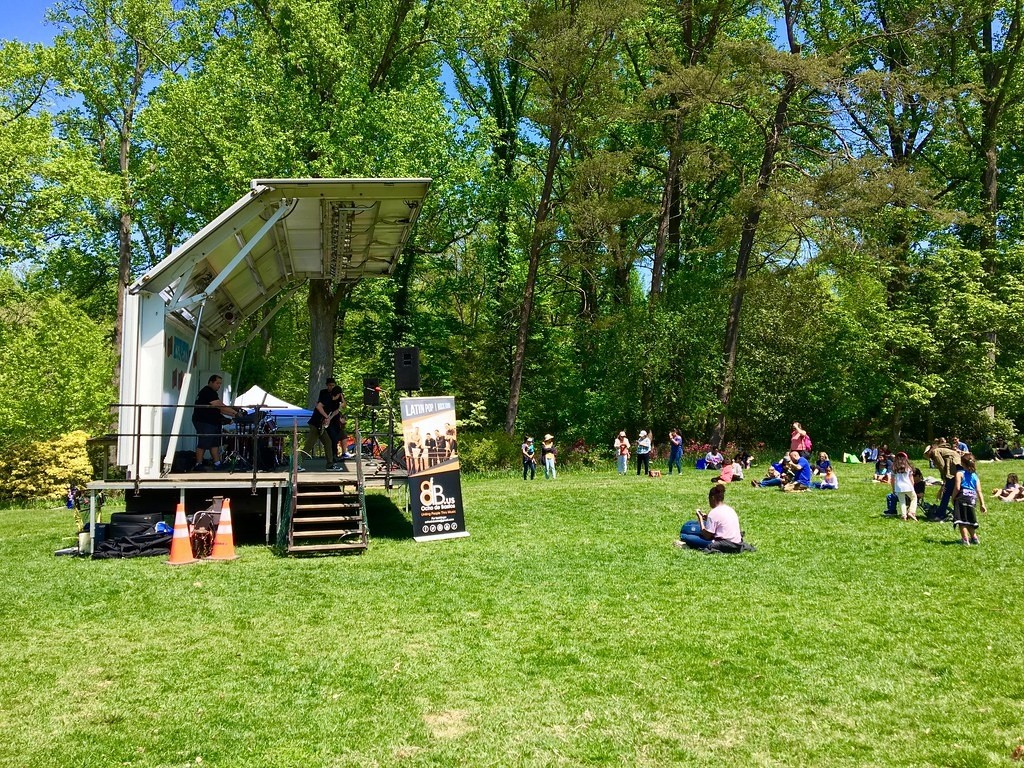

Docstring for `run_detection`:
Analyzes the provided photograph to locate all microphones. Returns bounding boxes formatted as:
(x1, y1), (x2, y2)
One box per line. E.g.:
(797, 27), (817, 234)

(375, 387), (388, 393)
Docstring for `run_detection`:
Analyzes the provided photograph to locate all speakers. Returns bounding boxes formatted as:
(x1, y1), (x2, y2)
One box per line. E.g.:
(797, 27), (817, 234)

(393, 346), (421, 391)
(363, 378), (380, 408)
(380, 444), (408, 470)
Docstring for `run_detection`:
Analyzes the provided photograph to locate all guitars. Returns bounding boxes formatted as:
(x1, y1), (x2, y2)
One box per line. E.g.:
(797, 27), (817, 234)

(319, 402), (346, 436)
(340, 410), (349, 427)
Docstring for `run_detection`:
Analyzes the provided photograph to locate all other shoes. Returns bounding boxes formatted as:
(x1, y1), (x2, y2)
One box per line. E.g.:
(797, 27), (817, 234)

(213, 463), (226, 470)
(667, 472), (672, 474)
(884, 509), (898, 515)
(678, 472), (682, 475)
(751, 480), (759, 487)
(962, 537), (979, 545)
(193, 463), (208, 472)
(900, 512), (918, 522)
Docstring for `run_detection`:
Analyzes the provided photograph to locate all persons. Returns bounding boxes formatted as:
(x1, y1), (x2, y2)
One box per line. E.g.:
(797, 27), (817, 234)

(522, 436), (537, 481)
(407, 423), (458, 474)
(790, 421), (806, 457)
(317, 379), (350, 459)
(192, 375), (243, 471)
(680, 484), (743, 553)
(666, 428), (682, 475)
(923, 436), (969, 521)
(705, 446), (754, 483)
(883, 452), (926, 520)
(296, 387), (344, 472)
(614, 431), (630, 475)
(951, 454), (987, 545)
(989, 473), (1024, 502)
(751, 449), (838, 492)
(222, 415), (235, 450)
(991, 437), (1024, 462)
(860, 443), (895, 483)
(636, 430), (651, 475)
(541, 434), (556, 479)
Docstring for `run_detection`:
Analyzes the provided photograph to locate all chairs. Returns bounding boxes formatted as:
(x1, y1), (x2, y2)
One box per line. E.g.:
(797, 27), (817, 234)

(906, 493), (927, 518)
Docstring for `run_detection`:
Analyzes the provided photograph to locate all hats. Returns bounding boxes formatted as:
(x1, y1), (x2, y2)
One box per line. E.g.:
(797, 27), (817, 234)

(620, 431), (626, 436)
(326, 378), (336, 385)
(527, 438), (532, 441)
(737, 447), (744, 452)
(544, 434), (554, 441)
(639, 430), (647, 437)
(923, 445), (933, 454)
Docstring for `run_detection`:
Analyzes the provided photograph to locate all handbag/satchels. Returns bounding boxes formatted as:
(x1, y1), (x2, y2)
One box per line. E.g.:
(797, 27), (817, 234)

(802, 451), (811, 459)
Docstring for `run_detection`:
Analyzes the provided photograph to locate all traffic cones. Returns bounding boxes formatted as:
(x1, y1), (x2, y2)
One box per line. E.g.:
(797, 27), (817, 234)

(161, 502), (200, 566)
(206, 498), (242, 561)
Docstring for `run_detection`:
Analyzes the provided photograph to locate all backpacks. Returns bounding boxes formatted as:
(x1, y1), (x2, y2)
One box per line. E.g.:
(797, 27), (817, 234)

(805, 433), (812, 450)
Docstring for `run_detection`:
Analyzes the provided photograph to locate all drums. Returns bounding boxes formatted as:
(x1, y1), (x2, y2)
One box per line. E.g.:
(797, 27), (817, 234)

(261, 418), (278, 433)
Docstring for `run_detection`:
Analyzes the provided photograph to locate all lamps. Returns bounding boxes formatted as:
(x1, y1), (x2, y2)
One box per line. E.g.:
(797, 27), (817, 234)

(223, 310), (236, 325)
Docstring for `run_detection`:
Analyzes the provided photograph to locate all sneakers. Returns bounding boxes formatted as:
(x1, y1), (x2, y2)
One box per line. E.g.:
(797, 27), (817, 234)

(341, 452), (356, 459)
(326, 462), (344, 472)
(297, 466), (305, 472)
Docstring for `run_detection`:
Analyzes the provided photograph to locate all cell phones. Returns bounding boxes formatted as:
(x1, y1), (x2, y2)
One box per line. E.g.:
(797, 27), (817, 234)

(696, 509), (704, 518)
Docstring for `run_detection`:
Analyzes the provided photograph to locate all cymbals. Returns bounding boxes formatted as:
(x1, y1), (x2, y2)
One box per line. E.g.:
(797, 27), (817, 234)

(249, 411), (266, 423)
(249, 405), (269, 408)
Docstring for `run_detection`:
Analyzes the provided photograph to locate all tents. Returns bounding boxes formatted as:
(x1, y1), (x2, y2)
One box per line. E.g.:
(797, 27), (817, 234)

(224, 385), (322, 457)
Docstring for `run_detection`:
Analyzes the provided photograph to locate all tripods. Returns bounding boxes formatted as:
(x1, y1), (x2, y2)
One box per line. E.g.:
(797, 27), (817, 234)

(221, 415), (252, 475)
(361, 407), (383, 459)
